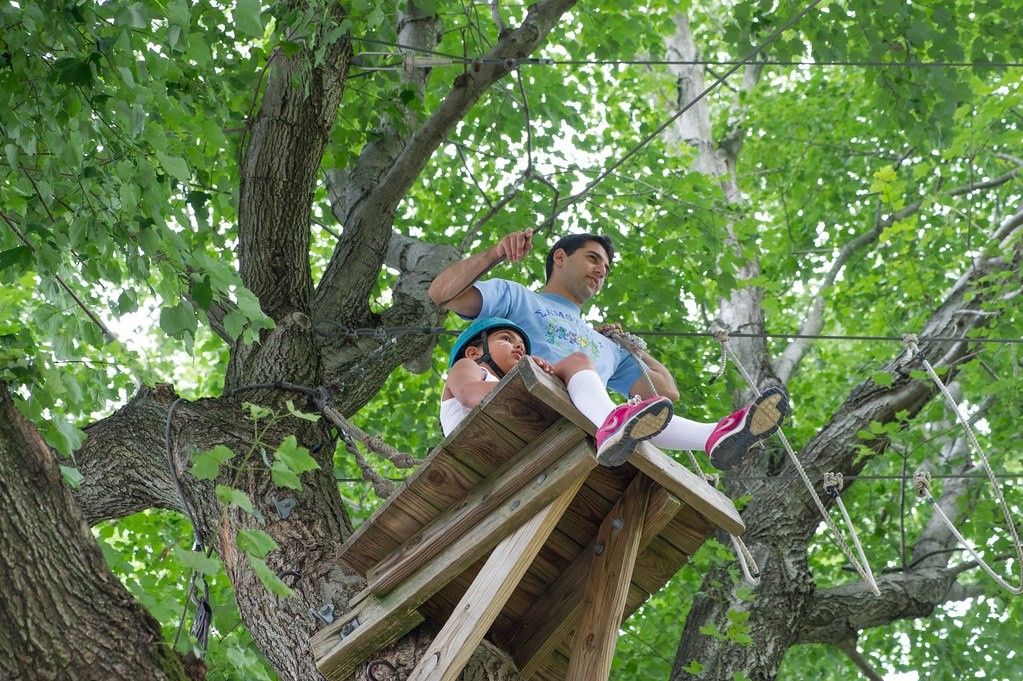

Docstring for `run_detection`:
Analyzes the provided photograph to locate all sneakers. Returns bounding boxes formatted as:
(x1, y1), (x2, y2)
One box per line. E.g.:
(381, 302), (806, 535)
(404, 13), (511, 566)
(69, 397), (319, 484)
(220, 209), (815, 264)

(705, 387), (789, 471)
(595, 395), (674, 467)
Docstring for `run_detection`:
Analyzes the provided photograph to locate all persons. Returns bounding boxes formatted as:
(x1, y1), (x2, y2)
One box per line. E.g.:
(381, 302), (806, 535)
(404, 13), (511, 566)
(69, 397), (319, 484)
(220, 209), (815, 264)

(438, 314), (790, 470)
(427, 226), (682, 407)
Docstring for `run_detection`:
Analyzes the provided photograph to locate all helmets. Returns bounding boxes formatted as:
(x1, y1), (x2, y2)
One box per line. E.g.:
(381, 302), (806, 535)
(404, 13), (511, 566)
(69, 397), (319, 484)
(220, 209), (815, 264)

(449, 317), (533, 368)
